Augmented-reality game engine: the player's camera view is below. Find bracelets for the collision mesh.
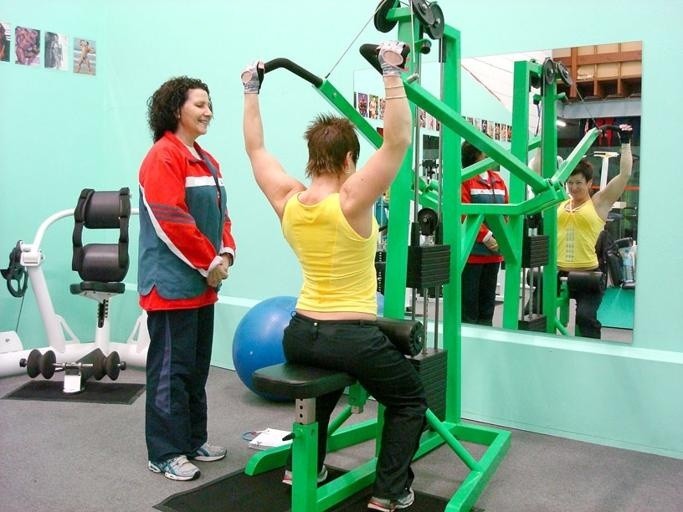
[384,95,407,100]
[385,84,402,89]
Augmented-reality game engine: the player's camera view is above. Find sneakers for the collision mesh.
[281,464,329,486]
[147,452,201,482]
[192,442,227,462]
[367,487,415,512]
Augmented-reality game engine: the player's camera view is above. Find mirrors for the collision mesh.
[353,40,642,347]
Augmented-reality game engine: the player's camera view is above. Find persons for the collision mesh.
[75,39,95,74]
[524,123,632,341]
[138,77,237,482]
[0,23,7,61]
[49,33,64,69]
[239,39,425,511]
[14,28,39,64]
[453,140,508,327]
